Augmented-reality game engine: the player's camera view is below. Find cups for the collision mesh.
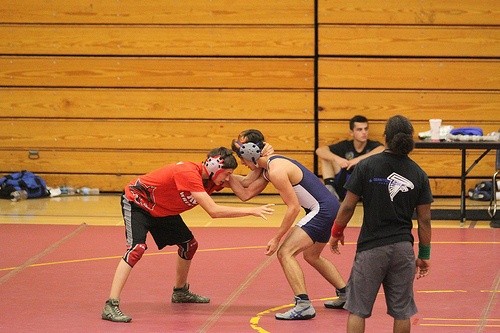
[429,119,442,141]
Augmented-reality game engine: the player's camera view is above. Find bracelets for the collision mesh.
[331,221,347,237]
[418,244,430,260]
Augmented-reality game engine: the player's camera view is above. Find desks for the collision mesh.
[415,140,500,223]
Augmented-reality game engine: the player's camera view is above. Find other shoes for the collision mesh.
[474,180,492,191]
[102,298,132,323]
[171,282,211,303]
[274,295,316,320]
[468,188,490,201]
[324,289,347,308]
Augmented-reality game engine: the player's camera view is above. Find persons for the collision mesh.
[101,142,275,323]
[314,114,386,202]
[333,115,433,333]
[227,129,348,319]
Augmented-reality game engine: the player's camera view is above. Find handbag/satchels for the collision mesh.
[0,169,51,199]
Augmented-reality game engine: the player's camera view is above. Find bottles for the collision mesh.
[56,187,75,195]
[76,187,99,195]
[8,189,28,201]
